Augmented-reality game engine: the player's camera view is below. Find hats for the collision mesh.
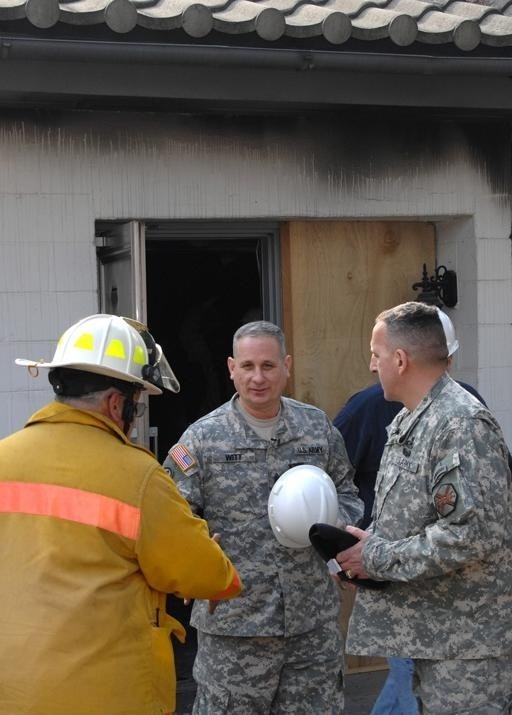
[309,524,392,590]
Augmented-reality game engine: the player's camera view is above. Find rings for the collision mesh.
[347,570,352,579]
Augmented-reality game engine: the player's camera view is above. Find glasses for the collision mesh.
[121,393,146,417]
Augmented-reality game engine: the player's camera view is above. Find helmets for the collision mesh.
[15,314,163,395]
[267,465,338,549]
[433,307,459,358]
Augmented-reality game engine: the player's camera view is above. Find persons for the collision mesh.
[337,301,512,714]
[333,305,490,715]
[160,322,365,715]
[0,314,244,715]
